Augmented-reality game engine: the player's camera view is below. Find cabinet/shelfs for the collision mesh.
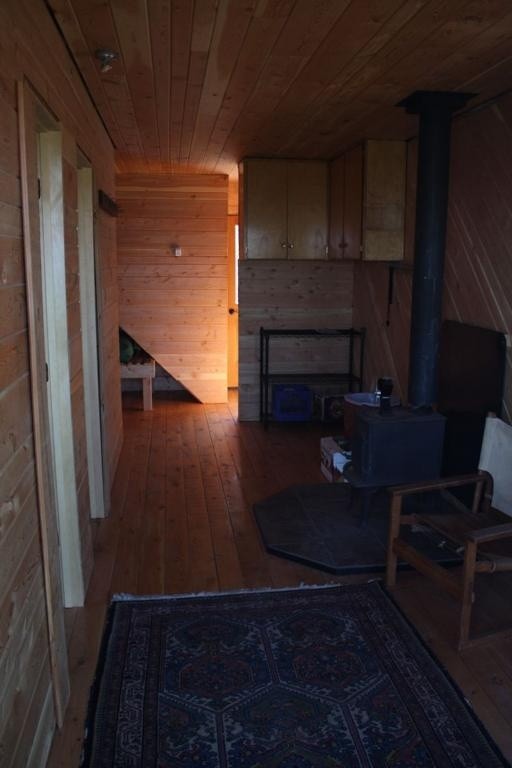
[257,325,366,431]
[330,137,408,262]
[238,156,330,259]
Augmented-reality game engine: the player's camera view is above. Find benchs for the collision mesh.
[121,356,156,411]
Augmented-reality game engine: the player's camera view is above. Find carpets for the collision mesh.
[78,576,509,767]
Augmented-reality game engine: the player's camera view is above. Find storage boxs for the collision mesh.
[319,436,352,482]
[313,389,345,424]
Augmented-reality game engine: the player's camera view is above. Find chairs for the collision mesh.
[383,411,512,651]
